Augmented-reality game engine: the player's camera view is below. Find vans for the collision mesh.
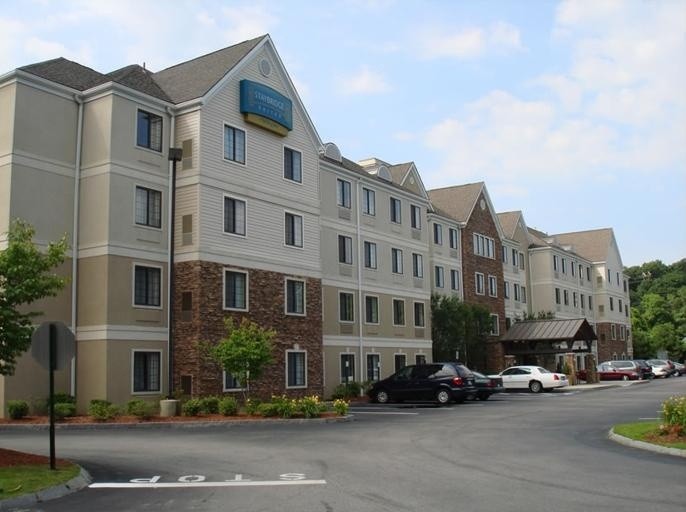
[366,361,478,407]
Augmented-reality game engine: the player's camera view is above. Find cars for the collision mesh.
[486,365,570,393]
[472,371,504,400]
[576,359,686,382]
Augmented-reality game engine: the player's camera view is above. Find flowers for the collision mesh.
[271,392,351,419]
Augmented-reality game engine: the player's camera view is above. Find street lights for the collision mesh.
[166,147,182,400]
